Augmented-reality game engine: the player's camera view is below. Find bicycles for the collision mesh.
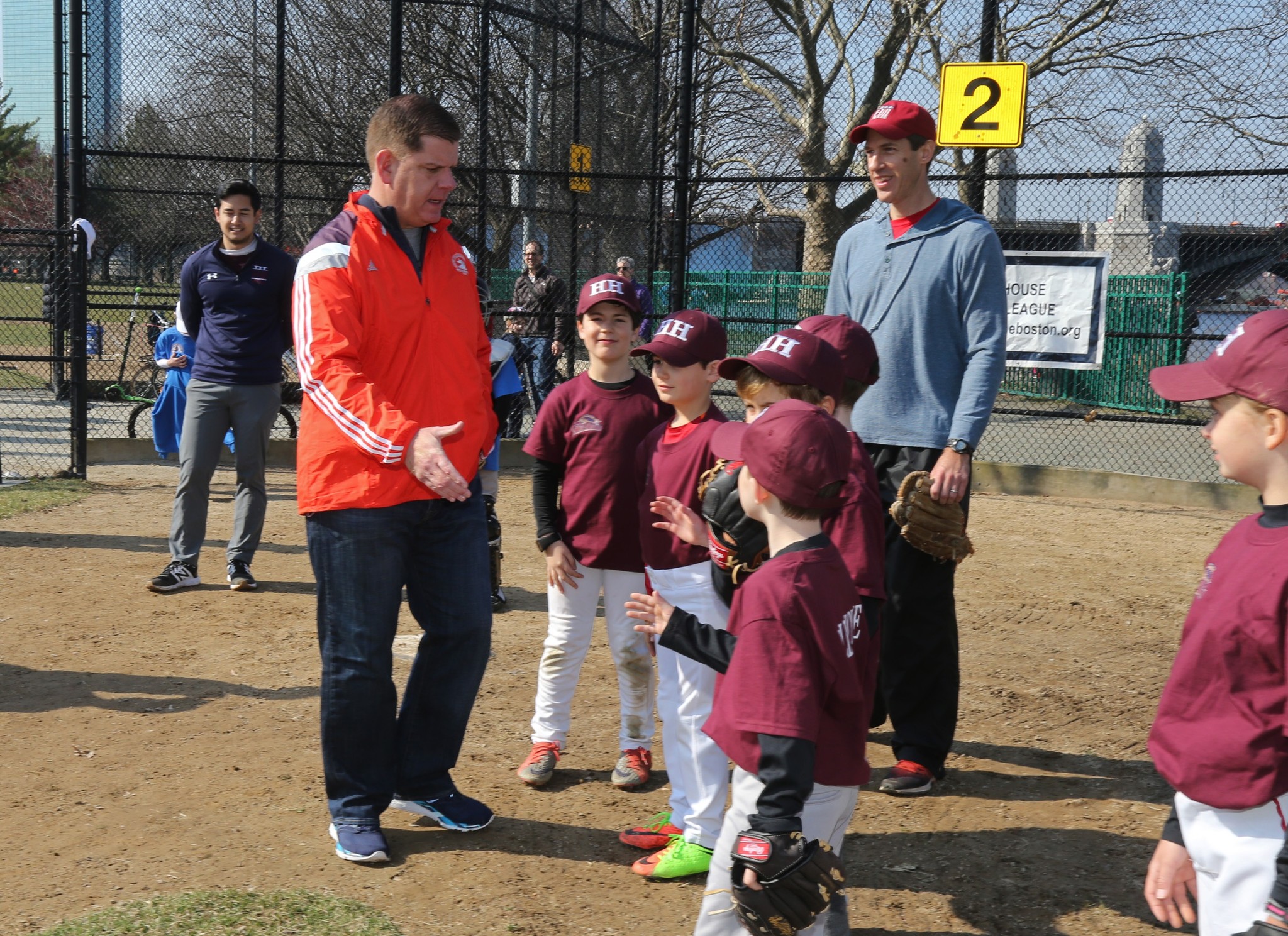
[127,302,297,438]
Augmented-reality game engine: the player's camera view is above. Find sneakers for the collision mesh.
[225,559,258,591]
[329,818,393,863]
[631,833,715,878]
[619,812,684,851]
[610,747,653,788]
[880,759,946,794]
[145,561,200,591]
[389,782,496,833]
[516,741,559,787]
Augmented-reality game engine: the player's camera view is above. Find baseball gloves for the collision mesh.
[730,829,844,936]
[891,469,975,566]
[702,459,769,607]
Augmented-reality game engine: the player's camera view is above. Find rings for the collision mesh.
[950,489,959,493]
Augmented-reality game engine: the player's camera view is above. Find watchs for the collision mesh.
[944,438,974,456]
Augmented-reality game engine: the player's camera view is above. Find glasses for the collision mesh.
[615,266,631,271]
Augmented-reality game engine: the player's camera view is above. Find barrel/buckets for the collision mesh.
[86,326,103,354]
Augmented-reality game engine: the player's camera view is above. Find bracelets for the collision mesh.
[537,532,561,553]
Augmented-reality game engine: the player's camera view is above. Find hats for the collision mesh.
[713,329,845,399]
[175,302,189,334]
[712,399,851,511]
[788,314,881,385]
[1147,308,1287,413]
[629,309,729,369]
[575,273,643,318]
[848,99,938,142]
[72,220,95,261]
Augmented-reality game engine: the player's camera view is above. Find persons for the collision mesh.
[1171,291,1200,365]
[506,241,571,438]
[822,99,1008,796]
[144,178,299,593]
[1143,804,1197,928]
[624,400,874,936]
[1147,308,1288,936]
[520,273,674,789]
[154,300,237,457]
[616,257,654,380]
[618,308,880,881]
[292,92,502,866]
